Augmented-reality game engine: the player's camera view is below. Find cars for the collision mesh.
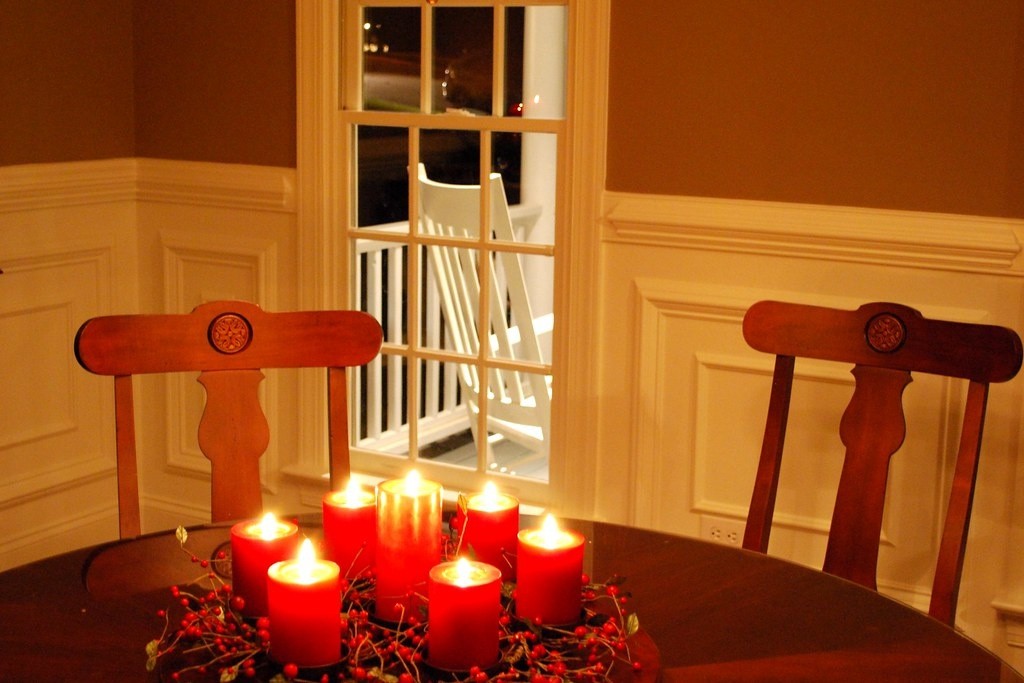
[441,46,524,117]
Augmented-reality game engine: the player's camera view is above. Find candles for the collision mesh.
[230,512,301,611]
[322,472,379,580]
[455,481,519,580]
[515,513,587,627]
[371,469,444,623]
[427,556,502,670]
[266,537,343,667]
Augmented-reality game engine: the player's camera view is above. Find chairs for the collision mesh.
[73,299,384,537]
[407,162,554,463]
[742,300,1024,625]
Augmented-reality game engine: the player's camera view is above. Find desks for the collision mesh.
[0,511,1024,683]
